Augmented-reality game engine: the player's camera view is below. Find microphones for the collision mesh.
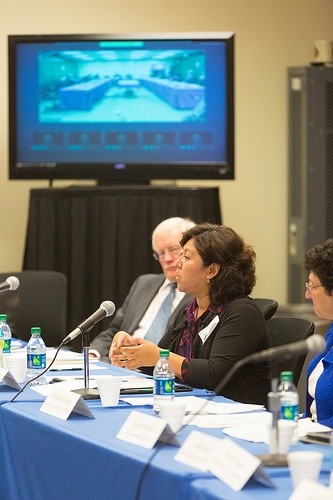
[62,301,116,344]
[240,334,326,369]
[0,276,20,292]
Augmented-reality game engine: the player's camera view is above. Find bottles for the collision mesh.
[0,314,12,369]
[153,349,175,415]
[0,327,5,369]
[275,372,299,445]
[27,327,46,386]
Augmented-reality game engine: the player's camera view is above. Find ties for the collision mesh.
[144,283,177,345]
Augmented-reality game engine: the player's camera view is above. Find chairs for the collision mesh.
[251,297,315,386]
[1,271,68,347]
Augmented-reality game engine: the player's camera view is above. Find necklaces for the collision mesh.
[196,307,199,319]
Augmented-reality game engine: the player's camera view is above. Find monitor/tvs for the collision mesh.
[8,34,235,187]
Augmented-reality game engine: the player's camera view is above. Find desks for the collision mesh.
[0,337,333,500]
[24,188,221,342]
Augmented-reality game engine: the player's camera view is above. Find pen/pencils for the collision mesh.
[49,368,82,371]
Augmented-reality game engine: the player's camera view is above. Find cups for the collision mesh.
[287,451,323,487]
[165,402,186,432]
[95,377,122,406]
[311,38,333,63]
[6,357,27,383]
[268,420,296,454]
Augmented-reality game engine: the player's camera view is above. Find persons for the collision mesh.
[107,222,276,412]
[84,217,202,381]
[302,235,333,430]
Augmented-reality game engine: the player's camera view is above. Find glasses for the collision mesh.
[153,246,181,260]
[306,283,329,294]
